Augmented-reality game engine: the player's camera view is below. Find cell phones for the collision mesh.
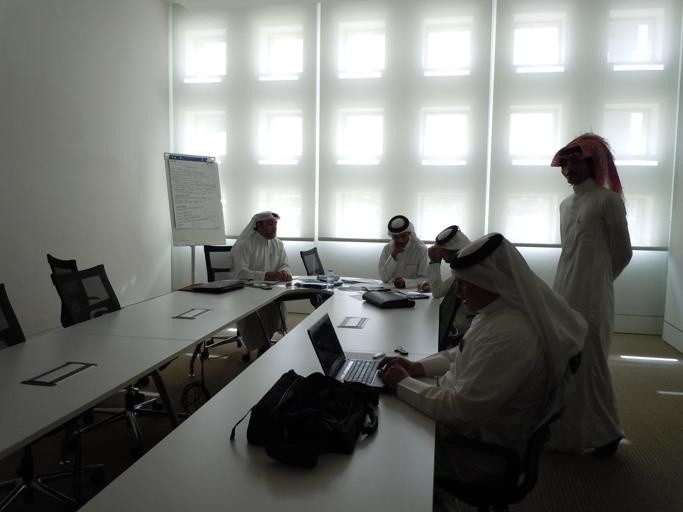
[408,294,429,299]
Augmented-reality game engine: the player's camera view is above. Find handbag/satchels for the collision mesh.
[246,369,381,454]
[362,290,414,308]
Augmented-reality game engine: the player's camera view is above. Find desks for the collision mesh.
[0,275,442,512]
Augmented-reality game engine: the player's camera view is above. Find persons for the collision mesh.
[549,133,632,456]
[230,212,292,357]
[430,226,473,299]
[378,215,429,289]
[376,233,587,511]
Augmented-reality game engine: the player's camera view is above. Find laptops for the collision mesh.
[307,313,388,391]
[192,280,244,293]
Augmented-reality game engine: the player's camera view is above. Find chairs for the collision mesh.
[189,243,250,374]
[45,251,190,440]
[298,247,331,306]
[0,279,83,511]
[435,345,583,510]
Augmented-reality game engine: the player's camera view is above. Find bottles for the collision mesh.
[326,269,334,291]
